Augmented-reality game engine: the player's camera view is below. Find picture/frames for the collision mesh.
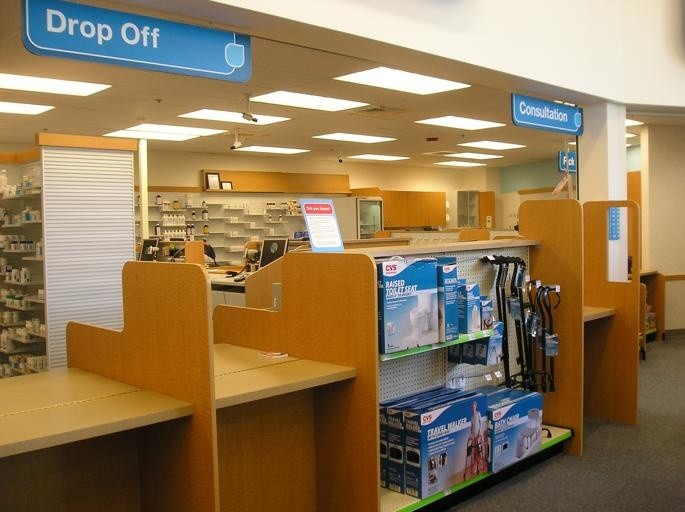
[221,181,233,189]
[206,172,221,190]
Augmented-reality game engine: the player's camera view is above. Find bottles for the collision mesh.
[0,169,47,377]
[137,194,302,241]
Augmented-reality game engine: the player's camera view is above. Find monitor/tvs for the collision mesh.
[139,238,158,261]
[259,238,289,270]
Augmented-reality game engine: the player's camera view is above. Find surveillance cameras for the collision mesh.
[242,113,258,122]
[336,153,343,164]
[230,141,242,149]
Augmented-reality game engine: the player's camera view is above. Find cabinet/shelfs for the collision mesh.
[0,132,138,378]
[0,191,640,511]
[628,270,666,358]
